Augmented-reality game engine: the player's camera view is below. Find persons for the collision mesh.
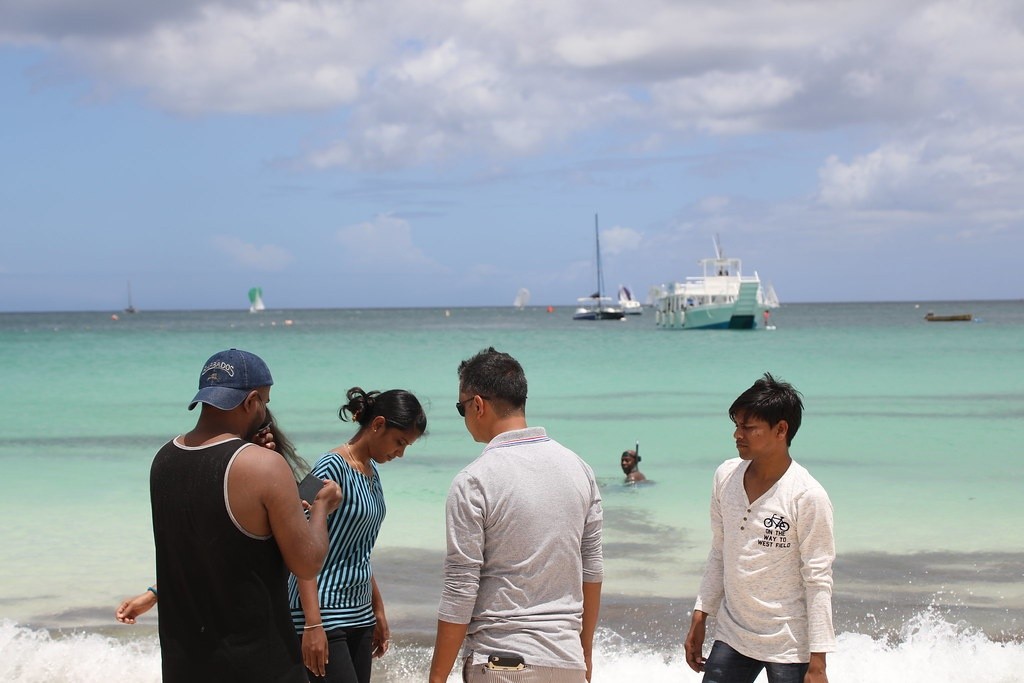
[684,372,837,683]
[720,265,728,276]
[763,310,769,326]
[429,347,603,683]
[116,347,344,683]
[286,387,427,683]
[620,450,646,483]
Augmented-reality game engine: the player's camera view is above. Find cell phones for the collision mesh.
[487,651,524,671]
[298,473,325,506]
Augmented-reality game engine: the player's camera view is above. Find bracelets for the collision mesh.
[304,622,324,629]
[148,586,157,595]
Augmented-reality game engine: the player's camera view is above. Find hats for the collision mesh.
[188,348,274,410]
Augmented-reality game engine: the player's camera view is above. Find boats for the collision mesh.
[925,312,974,322]
[654,235,780,330]
[616,285,644,316]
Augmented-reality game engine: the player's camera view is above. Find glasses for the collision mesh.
[456,396,491,417]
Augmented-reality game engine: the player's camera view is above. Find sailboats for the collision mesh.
[570,214,627,323]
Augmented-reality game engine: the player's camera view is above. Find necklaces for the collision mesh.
[345,442,373,476]
